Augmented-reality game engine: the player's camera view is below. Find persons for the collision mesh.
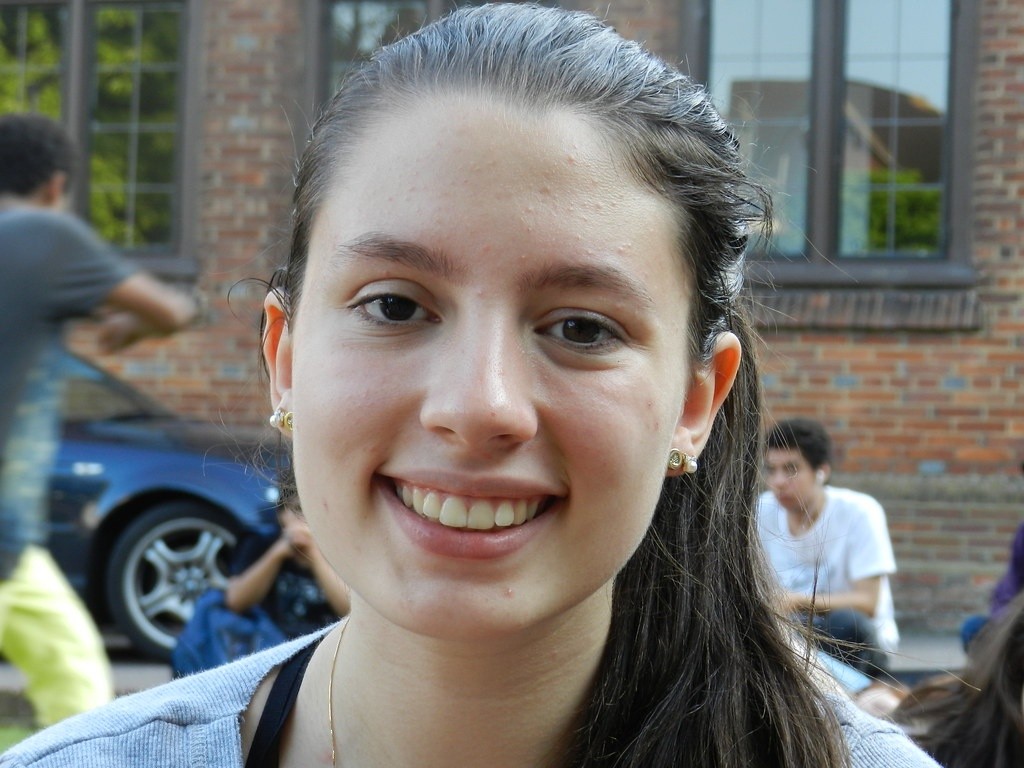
[1,2,944,768]
[746,420,900,676]
[0,107,196,736]
[895,453,1024,768]
[169,478,342,678]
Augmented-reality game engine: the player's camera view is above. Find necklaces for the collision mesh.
[327,618,349,766]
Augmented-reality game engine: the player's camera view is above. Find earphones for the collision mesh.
[815,471,826,491]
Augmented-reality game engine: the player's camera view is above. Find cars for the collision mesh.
[39,339,294,666]
